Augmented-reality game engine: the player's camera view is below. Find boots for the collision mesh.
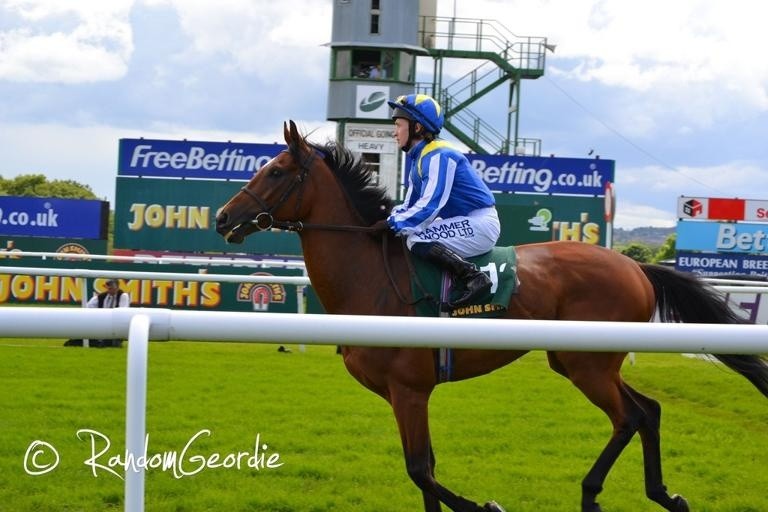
[422,242,493,307]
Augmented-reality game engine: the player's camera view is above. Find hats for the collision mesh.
[387,93,445,133]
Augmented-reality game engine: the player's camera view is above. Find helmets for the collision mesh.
[391,106,418,122]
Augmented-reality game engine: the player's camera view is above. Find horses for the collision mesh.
[214,120,767,512]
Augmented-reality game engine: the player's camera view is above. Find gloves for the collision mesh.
[368,219,392,236]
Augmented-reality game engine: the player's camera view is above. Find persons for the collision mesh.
[370,64,387,80]
[86,278,130,342]
[383,93,502,307]
